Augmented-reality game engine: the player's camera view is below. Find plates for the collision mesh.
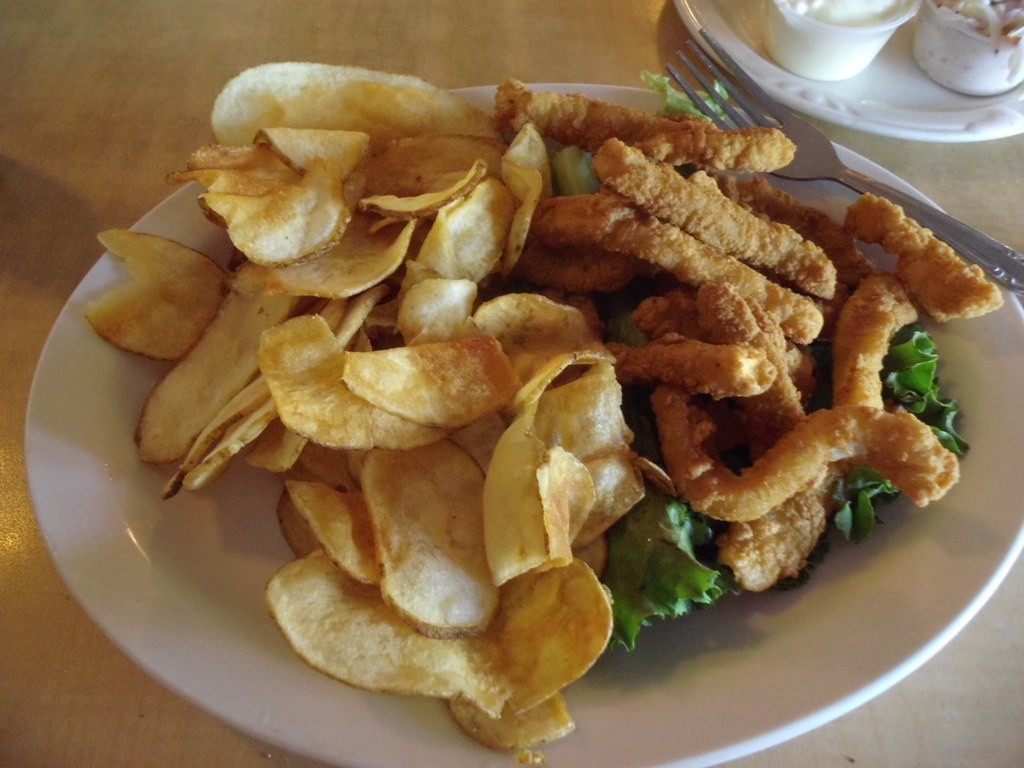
[675,0,1024,143]
[25,83,1024,768]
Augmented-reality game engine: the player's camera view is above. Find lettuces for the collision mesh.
[532,67,970,652]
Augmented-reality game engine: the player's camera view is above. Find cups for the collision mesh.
[763,0,923,82]
[912,0,1024,96]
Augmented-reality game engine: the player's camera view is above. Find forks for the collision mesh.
[664,27,1024,297]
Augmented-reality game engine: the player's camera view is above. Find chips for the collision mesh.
[85,60,647,757]
